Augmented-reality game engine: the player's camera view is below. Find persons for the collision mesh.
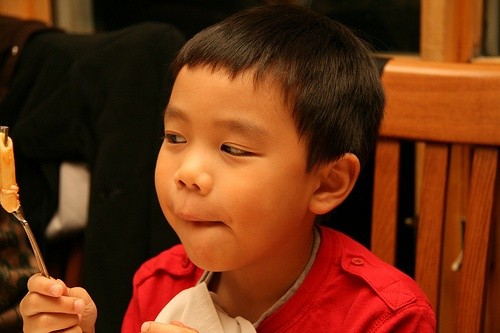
[19,2,437,332]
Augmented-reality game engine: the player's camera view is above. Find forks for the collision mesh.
[0,126,50,279]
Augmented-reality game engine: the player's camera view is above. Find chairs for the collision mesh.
[1,23,186,333]
[371,59,500,333]
